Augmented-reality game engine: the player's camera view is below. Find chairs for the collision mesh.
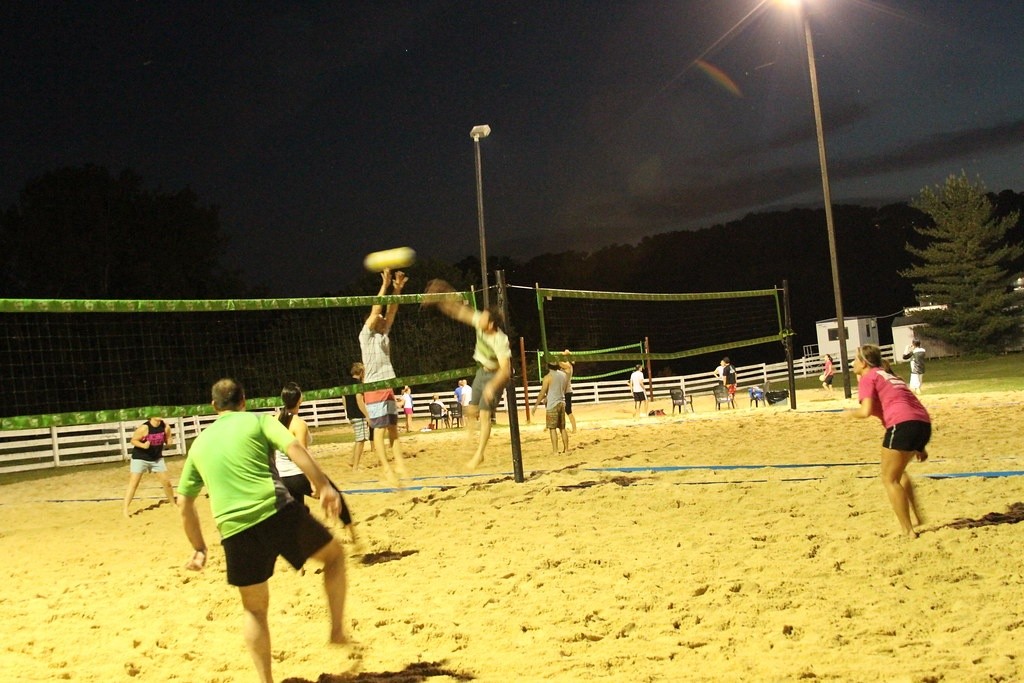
[429,403,450,430]
[712,385,734,412]
[449,401,464,428]
[750,381,771,407]
[670,388,695,415]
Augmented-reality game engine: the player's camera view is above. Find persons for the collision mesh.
[272,380,355,574]
[713,357,737,400]
[341,362,371,470]
[357,267,408,473]
[396,385,414,432]
[842,344,932,539]
[626,364,647,410]
[425,280,514,468]
[903,337,927,394]
[177,377,349,683]
[123,417,176,518]
[430,393,451,428]
[454,379,472,426]
[822,353,836,392]
[532,349,577,453]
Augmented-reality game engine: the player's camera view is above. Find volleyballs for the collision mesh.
[363,243,417,272]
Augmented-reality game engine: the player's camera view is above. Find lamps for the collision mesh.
[871,319,876,328]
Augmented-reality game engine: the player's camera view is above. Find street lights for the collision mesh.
[779,0,851,399]
[471,125,491,310]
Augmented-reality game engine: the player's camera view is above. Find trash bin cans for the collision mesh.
[766,389,789,406]
